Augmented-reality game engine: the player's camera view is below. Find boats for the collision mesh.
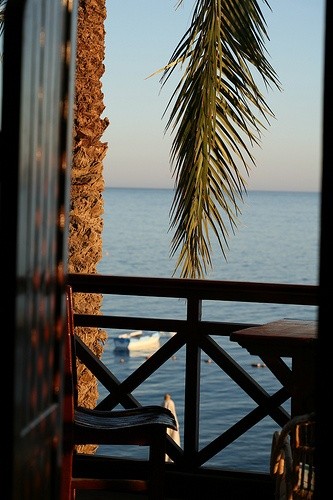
[113,330,160,349]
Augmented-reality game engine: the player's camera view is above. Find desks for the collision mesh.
[230,318,317,415]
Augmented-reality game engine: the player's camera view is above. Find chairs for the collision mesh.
[270,415,316,500]
[64,283,177,500]
